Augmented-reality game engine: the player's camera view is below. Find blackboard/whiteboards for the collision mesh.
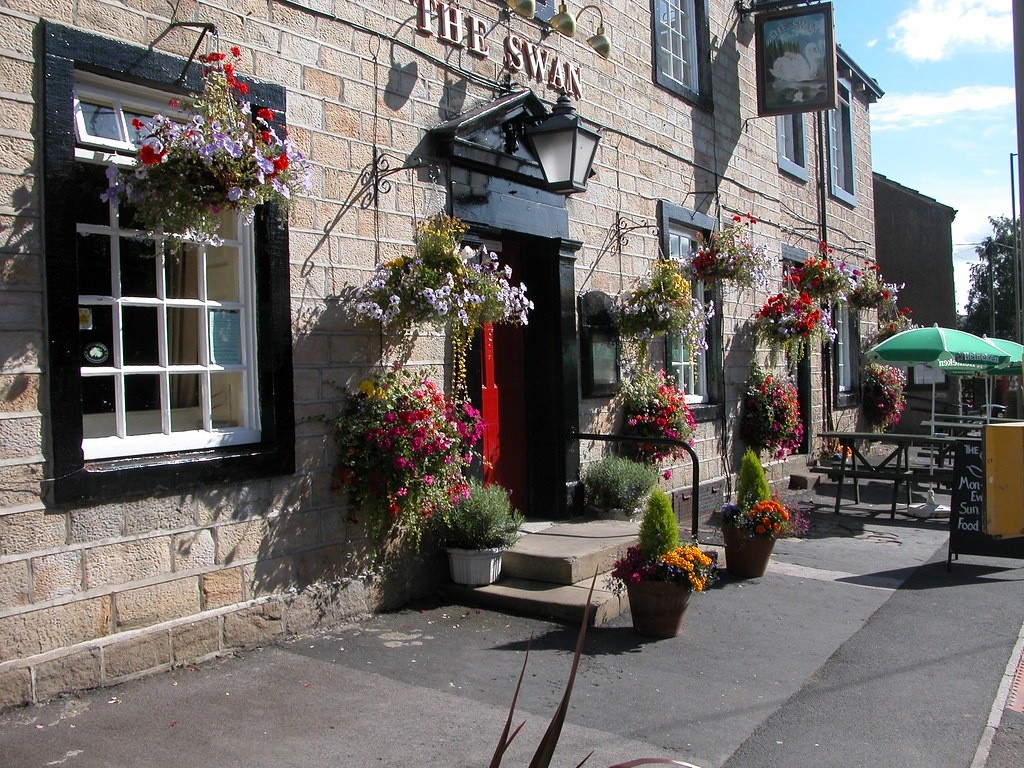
[950,440,1024,560]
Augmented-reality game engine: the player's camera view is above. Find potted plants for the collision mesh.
[423,474,527,585]
[577,454,662,520]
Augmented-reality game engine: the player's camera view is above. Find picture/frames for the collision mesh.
[755,1,838,118]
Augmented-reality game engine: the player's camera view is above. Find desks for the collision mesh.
[934,414,1023,437]
[920,418,984,467]
[818,430,982,518]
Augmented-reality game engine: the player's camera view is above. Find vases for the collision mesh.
[722,527,776,577]
[821,460,850,481]
[622,580,690,638]
[170,158,251,204]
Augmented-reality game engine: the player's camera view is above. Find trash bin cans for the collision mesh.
[980,404,1007,419]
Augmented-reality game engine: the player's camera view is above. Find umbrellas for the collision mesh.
[864,322,1024,518]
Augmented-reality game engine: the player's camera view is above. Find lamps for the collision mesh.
[517,86,602,194]
[503,0,611,59]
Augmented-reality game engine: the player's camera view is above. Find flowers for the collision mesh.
[607,213,924,479]
[601,488,720,596]
[713,446,814,547]
[99,47,314,263]
[295,213,516,591]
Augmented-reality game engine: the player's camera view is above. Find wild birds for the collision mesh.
[907,496,936,523]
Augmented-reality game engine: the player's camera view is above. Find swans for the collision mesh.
[768,42,819,83]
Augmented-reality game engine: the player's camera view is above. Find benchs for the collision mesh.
[809,447,958,482]
